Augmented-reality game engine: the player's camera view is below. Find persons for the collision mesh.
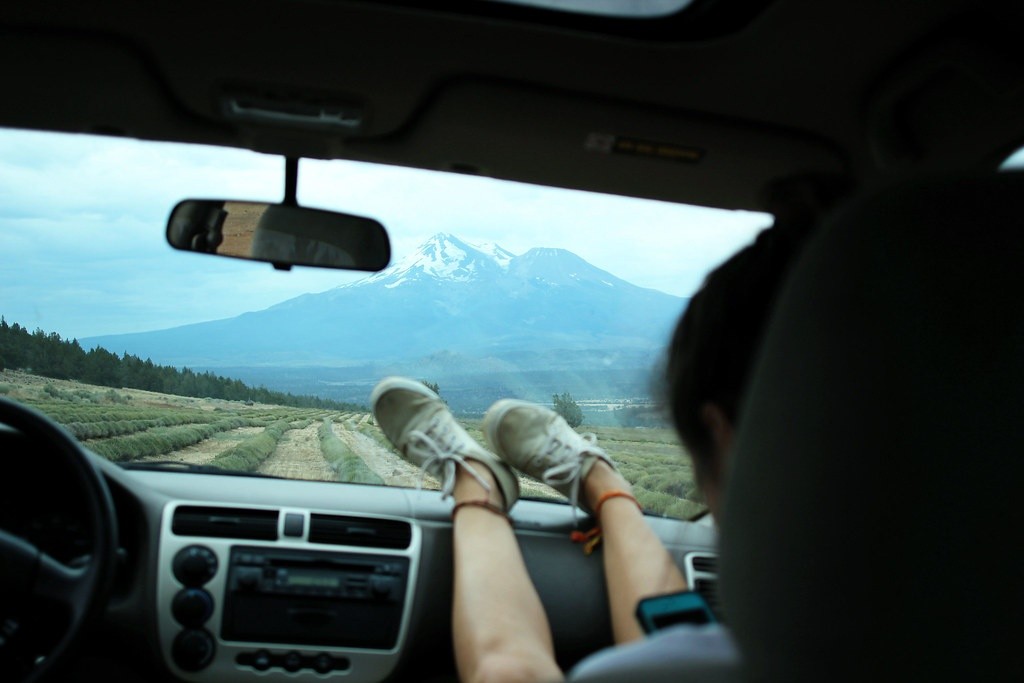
[371,218,822,683]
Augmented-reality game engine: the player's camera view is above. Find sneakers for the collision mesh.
[485,399,622,515]
[370,379,520,514]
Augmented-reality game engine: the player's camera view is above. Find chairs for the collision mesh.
[252,204,387,269]
[571,170,1024,683]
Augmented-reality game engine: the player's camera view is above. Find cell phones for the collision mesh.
[637,590,717,637]
